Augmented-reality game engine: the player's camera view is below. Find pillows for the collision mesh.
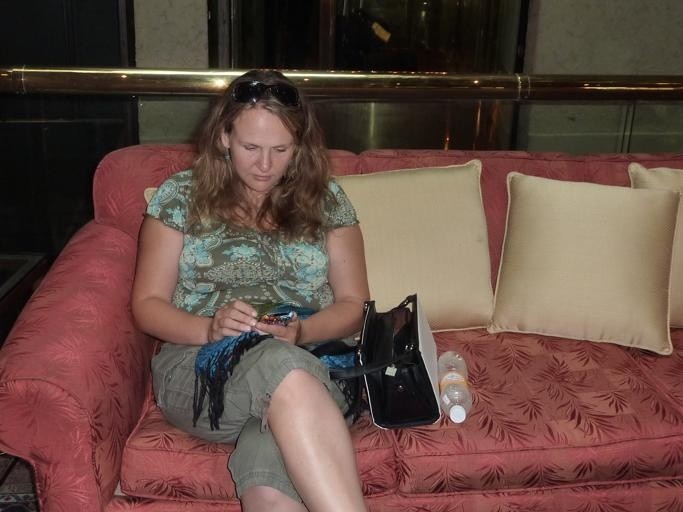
[488,171,680,355]
[329,160,494,333]
[627,162,681,328]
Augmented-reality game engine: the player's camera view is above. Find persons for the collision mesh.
[129,69,371,512]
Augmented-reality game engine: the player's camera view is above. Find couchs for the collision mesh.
[0,144,683,510]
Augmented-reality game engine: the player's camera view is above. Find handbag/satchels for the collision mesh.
[357,293,443,430]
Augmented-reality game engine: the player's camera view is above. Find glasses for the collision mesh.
[231,81,299,107]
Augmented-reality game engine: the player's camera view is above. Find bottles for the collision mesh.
[437,350,472,424]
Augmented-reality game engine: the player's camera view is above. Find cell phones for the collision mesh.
[259,312,294,328]
